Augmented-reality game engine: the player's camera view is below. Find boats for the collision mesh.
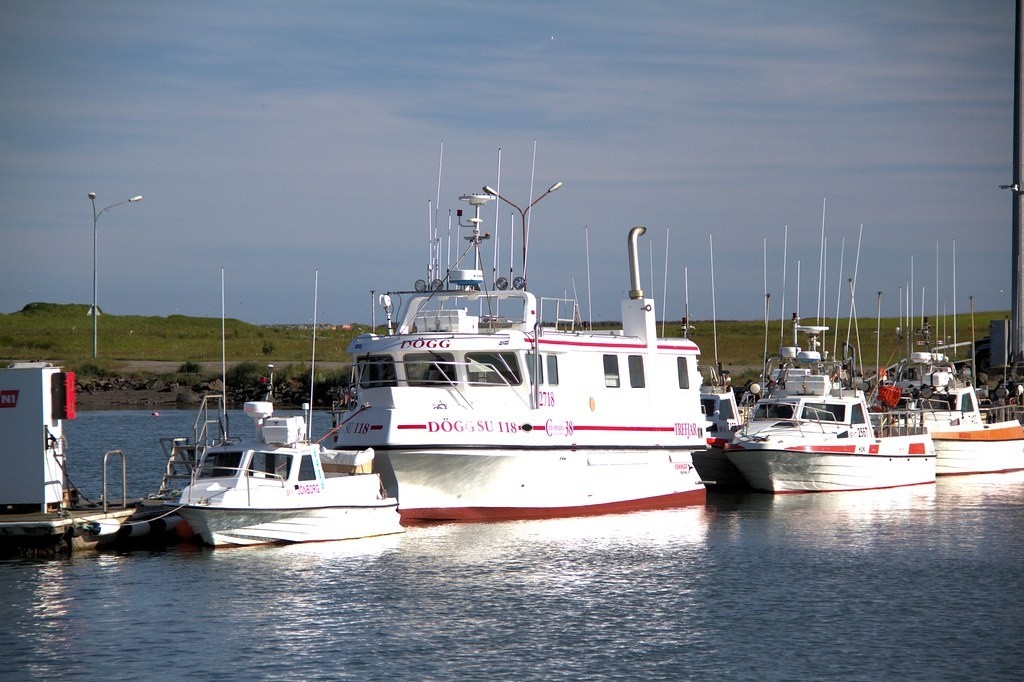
[336,195,716,521]
[892,386,1024,477]
[724,326,936,494]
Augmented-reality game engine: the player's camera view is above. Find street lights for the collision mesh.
[485,183,563,286]
[88,193,143,357]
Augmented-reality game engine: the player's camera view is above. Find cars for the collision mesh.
[177,418,406,551]
[969,336,1001,375]
